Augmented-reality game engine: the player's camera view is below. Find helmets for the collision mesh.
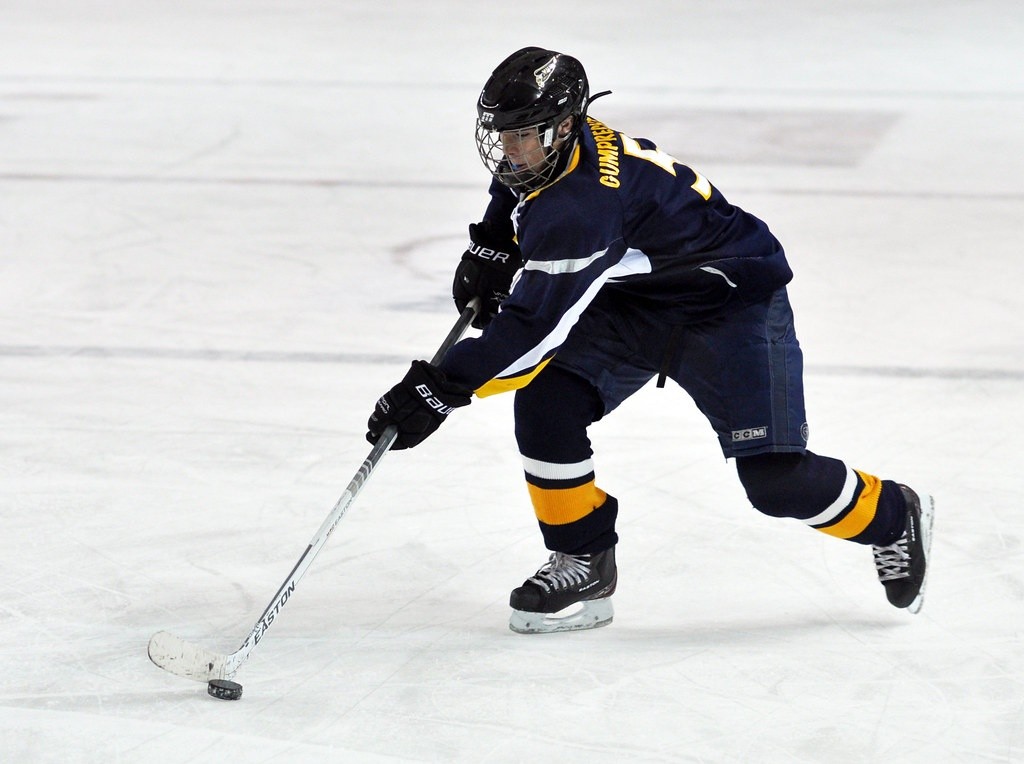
[475,46,589,191]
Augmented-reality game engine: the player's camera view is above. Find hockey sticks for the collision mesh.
[144,290,502,686]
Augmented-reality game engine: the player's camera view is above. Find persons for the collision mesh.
[366,47,934,634]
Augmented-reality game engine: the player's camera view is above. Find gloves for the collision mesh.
[453,224,523,329]
[366,360,473,450]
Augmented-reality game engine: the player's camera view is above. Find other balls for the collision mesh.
[207,679,243,701]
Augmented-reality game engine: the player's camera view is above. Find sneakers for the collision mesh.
[874,484,934,613]
[509,545,618,635]
